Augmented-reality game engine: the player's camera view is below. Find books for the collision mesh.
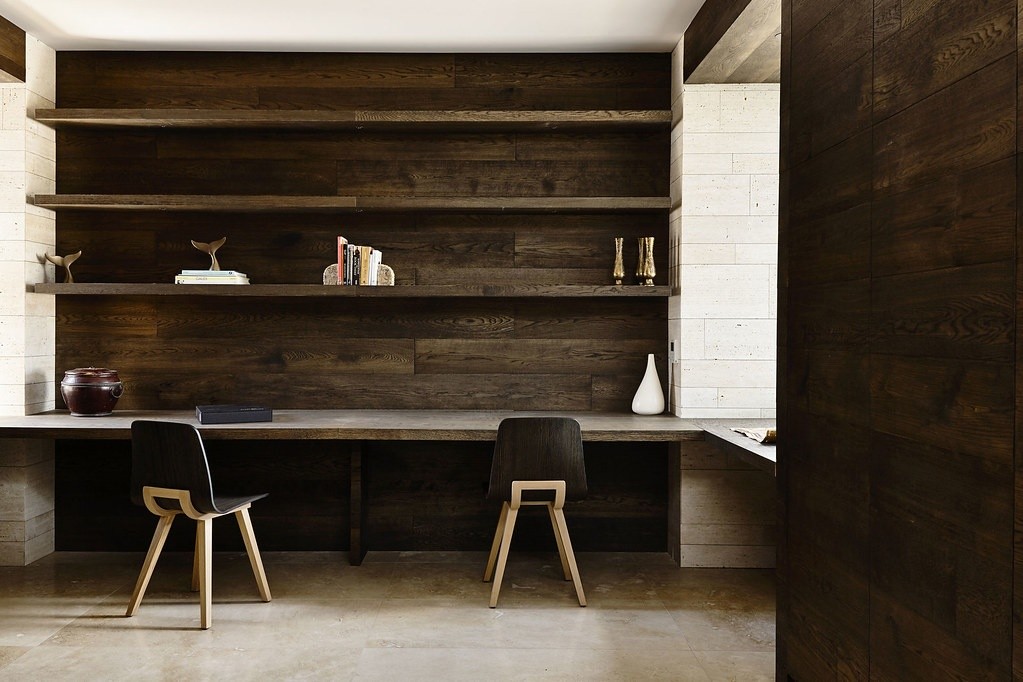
[174,270,250,284]
[195,406,272,425]
[338,237,382,285]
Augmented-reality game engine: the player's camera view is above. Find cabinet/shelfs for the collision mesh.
[33,107,675,298]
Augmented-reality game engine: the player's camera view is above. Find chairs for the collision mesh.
[482,416,588,607]
[125,421,272,630]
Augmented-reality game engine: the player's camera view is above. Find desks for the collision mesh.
[683,417,777,477]
[0,407,706,567]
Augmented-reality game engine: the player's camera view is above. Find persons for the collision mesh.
[355,247,360,255]
